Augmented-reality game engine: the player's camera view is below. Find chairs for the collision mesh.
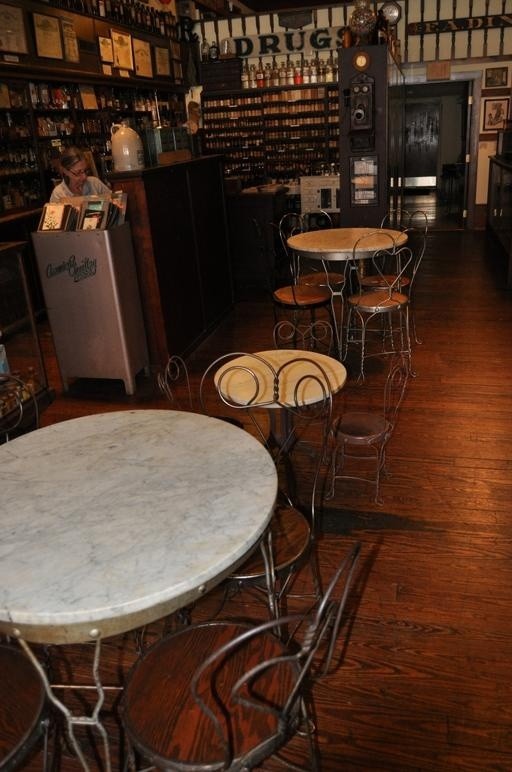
[2,349,362,771]
[156,321,411,519]
[274,210,428,384]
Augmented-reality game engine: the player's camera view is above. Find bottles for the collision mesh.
[0,117,118,213]
[46,0,179,40]
[209,41,219,61]
[240,57,339,89]
[201,40,210,63]
[220,39,232,59]
[211,147,337,182]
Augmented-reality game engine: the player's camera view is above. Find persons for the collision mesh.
[50,147,112,202]
[406,121,439,154]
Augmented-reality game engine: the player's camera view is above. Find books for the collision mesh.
[2,81,170,172]
[42,199,119,231]
[203,86,338,192]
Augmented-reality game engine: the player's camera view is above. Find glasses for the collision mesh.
[70,168,91,176]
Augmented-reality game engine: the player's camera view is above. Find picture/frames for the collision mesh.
[483,98,509,130]
[0,3,187,87]
[485,67,508,87]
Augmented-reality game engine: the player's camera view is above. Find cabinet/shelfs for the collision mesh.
[486,155,511,289]
[1,76,187,226]
[201,82,339,199]
[201,57,244,89]
[338,43,403,270]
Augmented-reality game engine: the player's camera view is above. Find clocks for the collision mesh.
[379,1,401,26]
